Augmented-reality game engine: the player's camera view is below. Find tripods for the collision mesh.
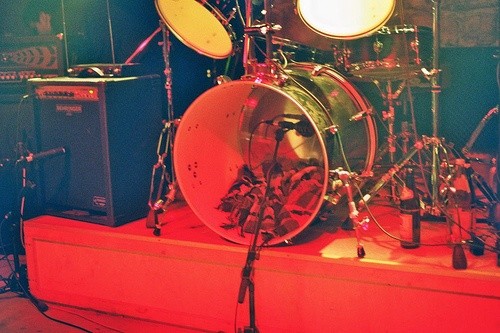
[342,1,499,231]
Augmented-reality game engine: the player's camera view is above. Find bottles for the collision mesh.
[399,165,421,248]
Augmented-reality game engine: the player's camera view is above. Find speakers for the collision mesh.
[27,71,164,228]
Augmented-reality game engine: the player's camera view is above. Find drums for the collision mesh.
[154,0,265,60]
[296,0,400,41]
[170,62,380,247]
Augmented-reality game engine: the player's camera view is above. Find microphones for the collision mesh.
[268,119,314,137]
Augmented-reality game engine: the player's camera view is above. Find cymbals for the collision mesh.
[353,67,420,82]
